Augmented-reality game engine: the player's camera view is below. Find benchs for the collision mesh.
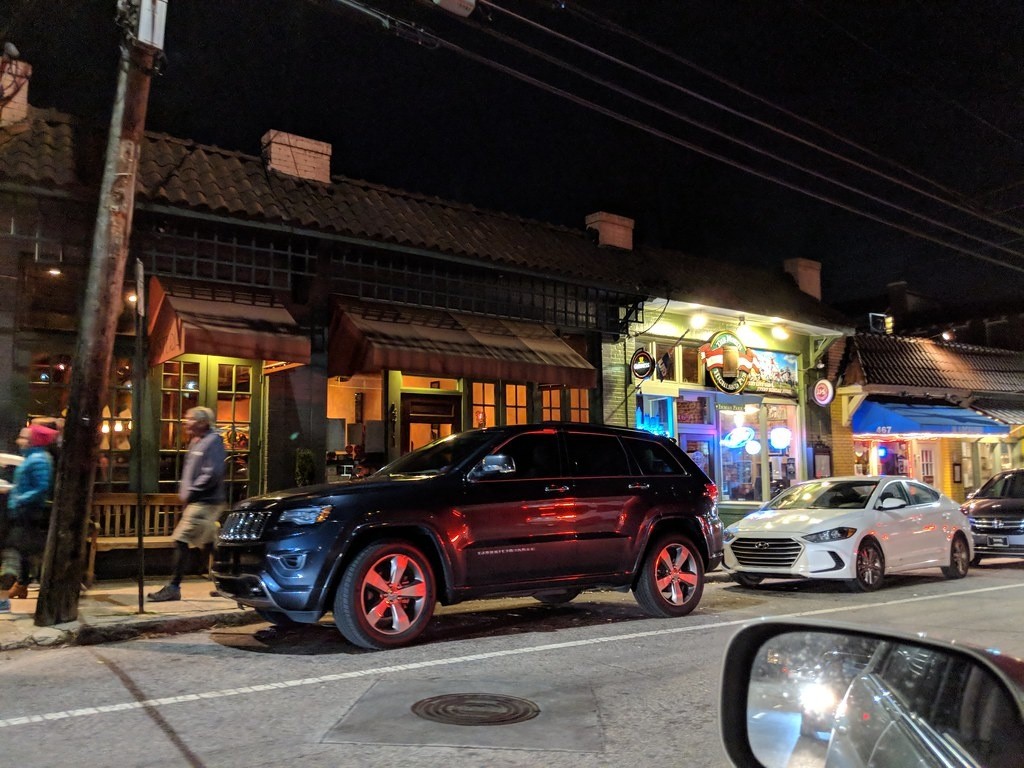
[82,492,227,584]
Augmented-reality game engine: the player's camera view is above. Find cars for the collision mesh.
[720,622,1024,768]
[723,474,975,593]
[795,652,873,737]
[959,467,1024,566]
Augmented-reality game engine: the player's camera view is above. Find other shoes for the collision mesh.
[8,582,28,599]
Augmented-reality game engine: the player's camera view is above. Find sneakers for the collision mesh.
[210,591,222,597]
[148,583,181,601]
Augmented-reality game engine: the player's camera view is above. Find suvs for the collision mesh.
[207,421,726,650]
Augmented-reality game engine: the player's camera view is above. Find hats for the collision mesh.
[28,424,59,446]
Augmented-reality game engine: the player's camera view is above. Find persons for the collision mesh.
[0,424,60,613]
[7,416,59,600]
[146,405,226,602]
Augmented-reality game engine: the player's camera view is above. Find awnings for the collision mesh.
[326,300,599,391]
[850,394,1011,439]
[968,400,1024,426]
[145,275,313,375]
